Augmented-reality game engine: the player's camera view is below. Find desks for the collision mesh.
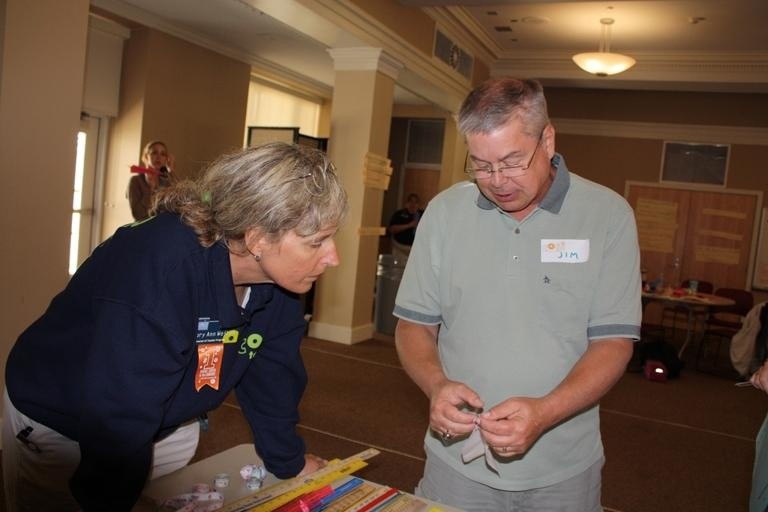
[642,282,736,362]
[141,442,466,512]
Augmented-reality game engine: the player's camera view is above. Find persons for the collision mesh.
[393,74,643,512]
[6,142,346,511]
[748,304,768,511]
[125,142,178,221]
[388,193,424,255]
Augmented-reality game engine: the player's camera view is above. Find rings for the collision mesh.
[503,447,507,453]
[443,431,451,441]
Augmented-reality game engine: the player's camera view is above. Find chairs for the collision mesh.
[659,278,754,371]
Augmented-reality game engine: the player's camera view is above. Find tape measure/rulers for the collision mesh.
[214,447,428,512]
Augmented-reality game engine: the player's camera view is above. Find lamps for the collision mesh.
[571,18,637,76]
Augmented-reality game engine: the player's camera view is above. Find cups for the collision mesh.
[689,280,698,293]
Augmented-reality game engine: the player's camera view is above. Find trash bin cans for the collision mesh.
[376,252,407,337]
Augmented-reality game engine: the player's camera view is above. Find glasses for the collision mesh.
[462,128,546,179]
[280,153,337,197]
